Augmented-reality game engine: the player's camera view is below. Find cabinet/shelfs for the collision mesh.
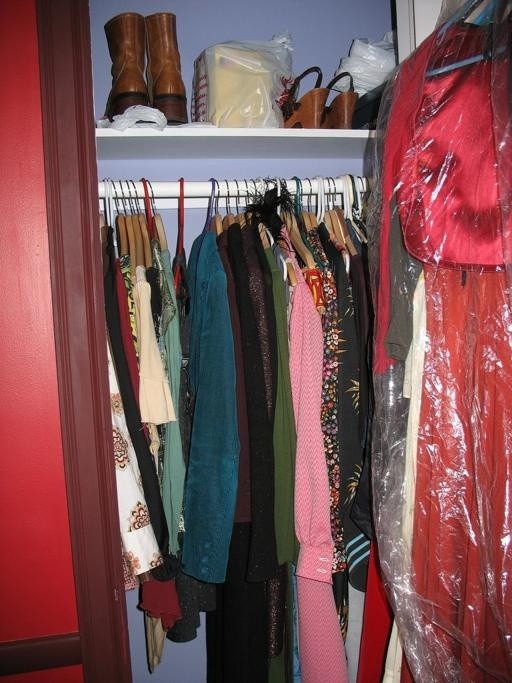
[0,2,448,682]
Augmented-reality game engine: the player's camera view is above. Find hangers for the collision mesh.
[96,174,370,289]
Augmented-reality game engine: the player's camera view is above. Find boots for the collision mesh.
[103,11,188,124]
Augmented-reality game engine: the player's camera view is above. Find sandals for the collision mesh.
[281,66,359,129]
